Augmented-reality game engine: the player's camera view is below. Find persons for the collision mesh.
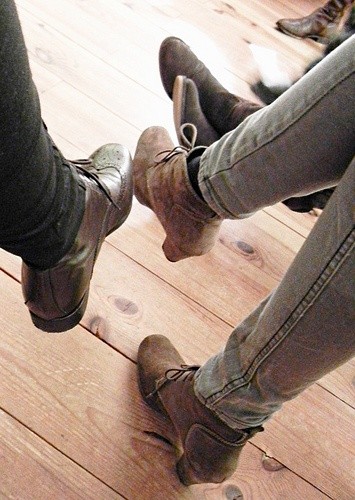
[133,31,355,486]
[160,0,355,215]
[0,0,133,334]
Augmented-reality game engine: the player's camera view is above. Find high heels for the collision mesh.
[131,126,223,262]
[136,334,264,487]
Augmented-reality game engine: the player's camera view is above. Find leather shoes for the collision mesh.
[159,37,236,150]
[21,143,132,332]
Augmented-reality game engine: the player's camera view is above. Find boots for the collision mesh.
[275,0,347,45]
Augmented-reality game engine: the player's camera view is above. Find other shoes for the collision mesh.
[250,80,290,106]
